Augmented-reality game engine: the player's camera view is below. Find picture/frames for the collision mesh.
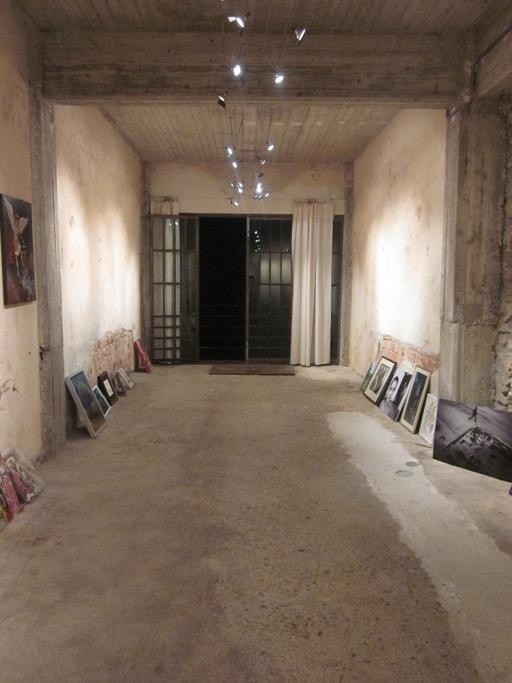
[0,192,38,308]
[360,353,439,445]
[63,362,137,438]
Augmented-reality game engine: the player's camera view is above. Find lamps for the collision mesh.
[213,0,311,206]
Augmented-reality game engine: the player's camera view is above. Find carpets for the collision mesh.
[209,362,296,375]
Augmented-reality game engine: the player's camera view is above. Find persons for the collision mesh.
[379,375,399,421]
